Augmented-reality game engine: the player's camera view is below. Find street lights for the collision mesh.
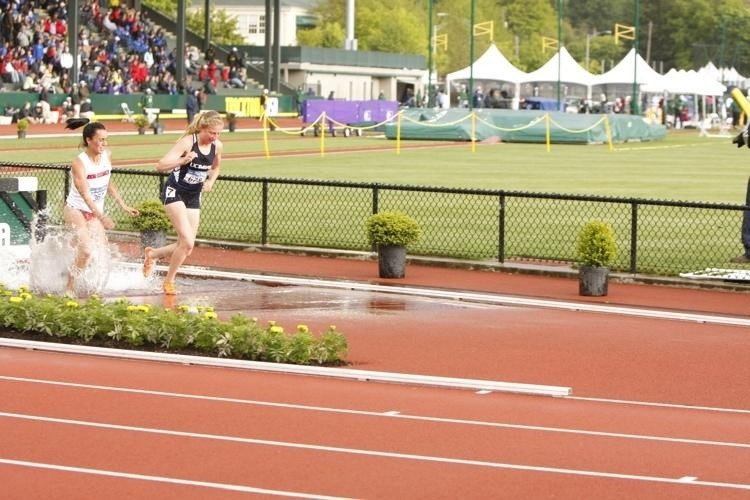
[432,11,446,76]
[583,28,614,72]
[503,21,520,69]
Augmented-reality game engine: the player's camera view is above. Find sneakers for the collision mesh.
[163,278,175,295]
[143,248,153,278]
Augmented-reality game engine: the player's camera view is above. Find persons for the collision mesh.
[258,89,270,122]
[0,0,251,126]
[730,120,750,263]
[295,84,304,114]
[62,116,141,300]
[143,109,225,295]
[728,99,743,126]
[402,83,721,131]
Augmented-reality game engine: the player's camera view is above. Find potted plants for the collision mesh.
[364,210,421,278]
[128,200,176,259]
[572,217,618,296]
